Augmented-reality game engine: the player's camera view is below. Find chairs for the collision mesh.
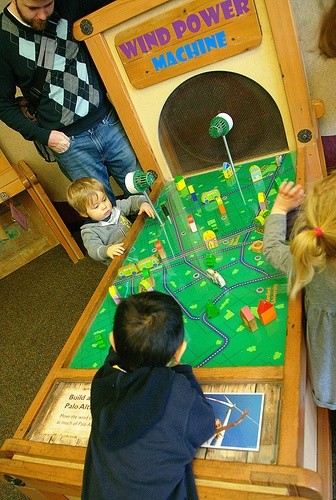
[0,149,85,279]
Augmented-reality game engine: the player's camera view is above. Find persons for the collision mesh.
[263,161,336,456]
[0,0,148,208]
[214,409,248,438]
[80,291,215,500]
[67,177,155,266]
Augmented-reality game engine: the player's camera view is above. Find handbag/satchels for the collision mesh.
[16,95,56,163]
